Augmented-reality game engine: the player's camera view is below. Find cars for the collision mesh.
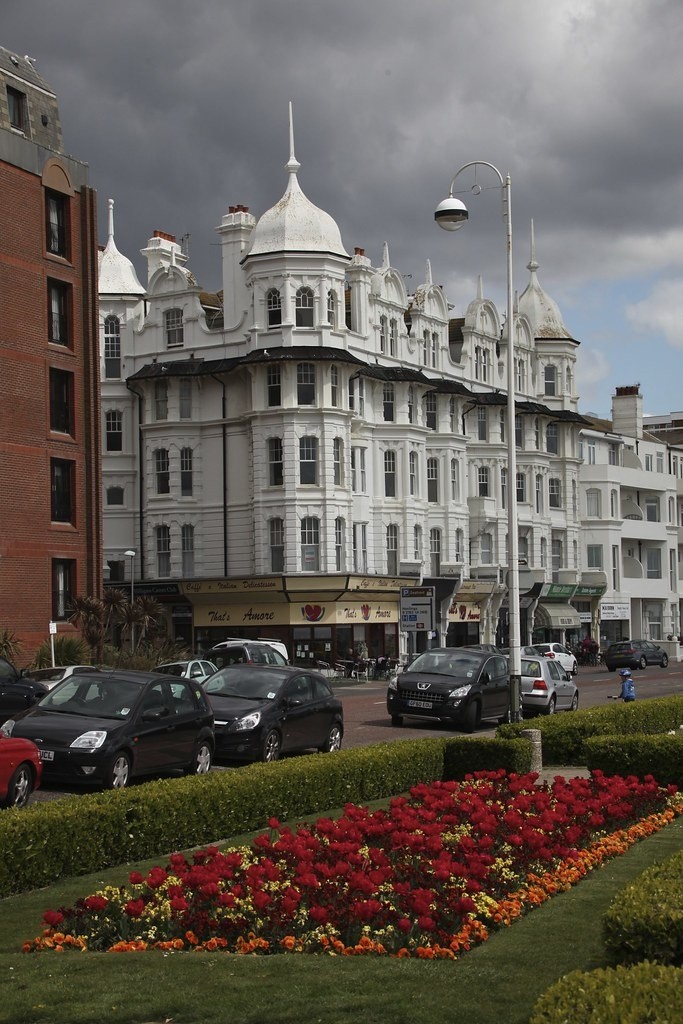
[0,654,50,724]
[520,656,579,715]
[199,664,344,764]
[529,643,579,676]
[498,646,541,658]
[1,669,216,793]
[28,664,107,706]
[461,644,502,654]
[0,736,43,810]
[148,660,225,699]
[386,647,510,733]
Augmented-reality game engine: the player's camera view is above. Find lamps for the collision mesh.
[263,350,270,358]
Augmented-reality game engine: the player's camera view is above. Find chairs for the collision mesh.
[316,659,407,683]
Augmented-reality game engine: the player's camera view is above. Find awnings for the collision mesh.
[534,603,581,630]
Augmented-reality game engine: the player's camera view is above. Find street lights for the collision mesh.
[432,159,522,723]
[123,550,136,656]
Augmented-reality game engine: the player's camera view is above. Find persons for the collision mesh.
[583,635,600,665]
[618,669,635,702]
[345,648,355,678]
[378,655,390,677]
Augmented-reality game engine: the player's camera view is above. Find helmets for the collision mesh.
[618,669,631,676]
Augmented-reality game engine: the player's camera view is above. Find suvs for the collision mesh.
[203,641,289,672]
[606,639,669,672]
